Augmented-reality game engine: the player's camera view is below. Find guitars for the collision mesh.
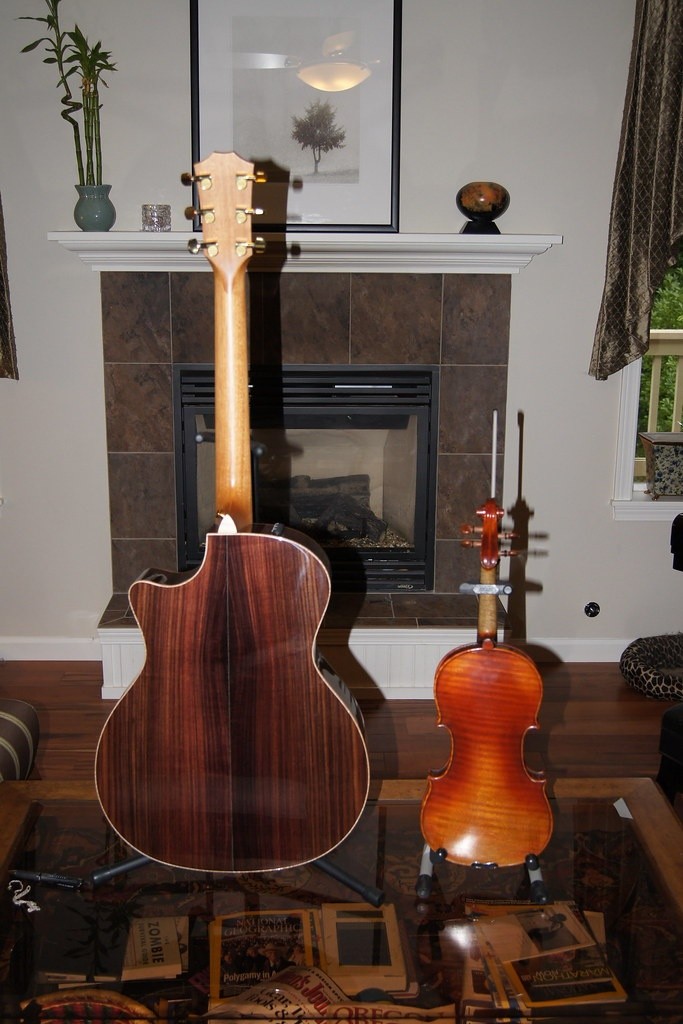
[76,152,384,906]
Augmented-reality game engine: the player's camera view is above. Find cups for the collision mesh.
[142,205,171,232]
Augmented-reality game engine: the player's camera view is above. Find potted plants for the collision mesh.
[15,0,117,230]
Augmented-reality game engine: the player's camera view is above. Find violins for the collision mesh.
[415,408,554,902]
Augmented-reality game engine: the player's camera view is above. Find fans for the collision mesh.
[207,28,399,79]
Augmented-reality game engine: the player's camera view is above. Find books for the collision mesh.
[36,902,628,1024]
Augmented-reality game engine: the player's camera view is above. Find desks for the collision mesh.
[0,776,683,1024]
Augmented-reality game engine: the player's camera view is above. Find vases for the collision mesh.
[639,432,683,500]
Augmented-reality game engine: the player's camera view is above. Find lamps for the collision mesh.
[297,60,372,93]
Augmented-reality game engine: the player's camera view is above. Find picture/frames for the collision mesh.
[190,0,404,234]
